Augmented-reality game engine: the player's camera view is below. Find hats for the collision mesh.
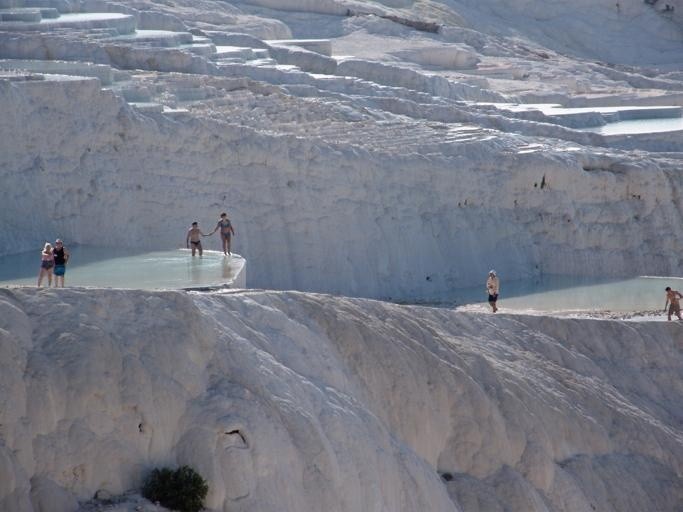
[488,269,497,277]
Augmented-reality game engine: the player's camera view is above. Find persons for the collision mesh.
[661,286,682,321]
[37,242,53,287]
[486,270,499,312]
[51,238,66,288]
[209,211,234,257]
[185,221,209,256]
